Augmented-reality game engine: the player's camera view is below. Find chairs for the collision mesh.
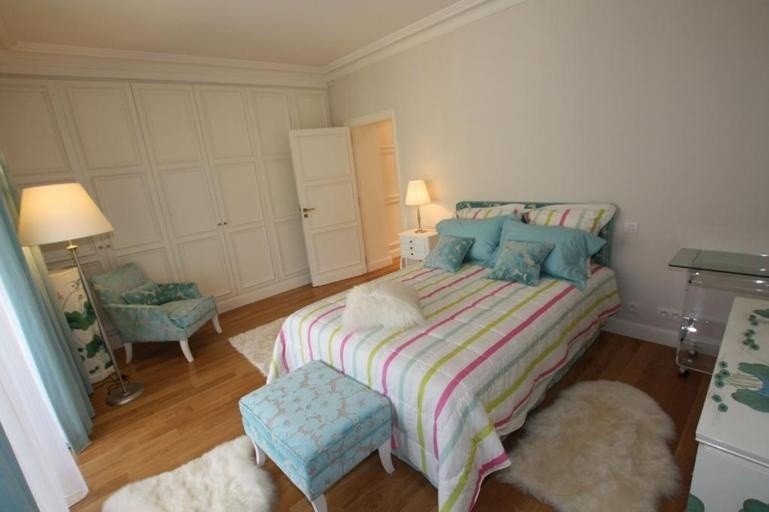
[89,263,223,363]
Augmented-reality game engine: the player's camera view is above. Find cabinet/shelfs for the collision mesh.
[1,76,183,353]
[247,78,339,292]
[685,293,769,512]
[130,78,286,318]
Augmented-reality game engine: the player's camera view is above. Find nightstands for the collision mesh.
[398,228,439,270]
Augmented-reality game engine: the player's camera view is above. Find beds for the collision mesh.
[265,200,622,512]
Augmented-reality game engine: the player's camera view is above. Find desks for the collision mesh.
[667,247,769,379]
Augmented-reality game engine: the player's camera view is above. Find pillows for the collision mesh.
[424,204,618,291]
[339,275,425,334]
[120,278,166,307]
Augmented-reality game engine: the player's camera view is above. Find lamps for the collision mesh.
[405,180,432,234]
[16,182,146,406]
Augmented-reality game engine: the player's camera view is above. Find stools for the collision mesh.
[238,361,396,511]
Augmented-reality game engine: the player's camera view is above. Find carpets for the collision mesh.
[228,316,288,377]
[494,380,685,511]
[100,434,279,511]
